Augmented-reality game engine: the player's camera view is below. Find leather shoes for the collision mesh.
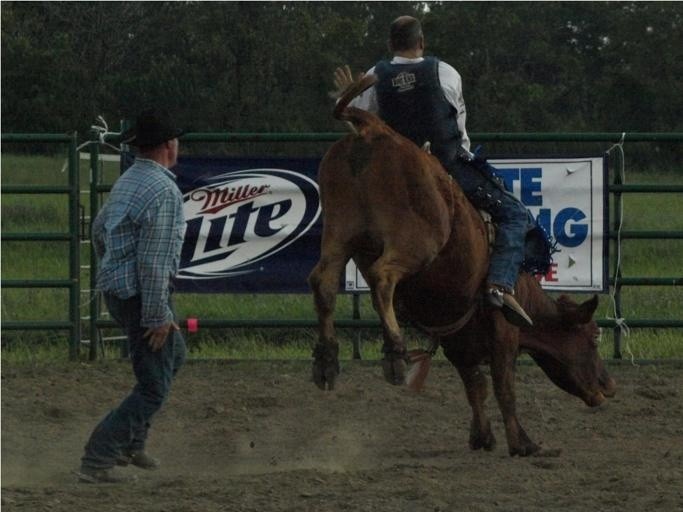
[123,449,161,471]
[484,283,532,329]
[79,470,138,485]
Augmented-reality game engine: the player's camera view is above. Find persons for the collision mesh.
[333,16,535,330]
[81,111,193,483]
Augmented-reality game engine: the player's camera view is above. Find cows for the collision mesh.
[307,64,617,457]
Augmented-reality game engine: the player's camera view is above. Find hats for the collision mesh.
[125,109,184,145]
